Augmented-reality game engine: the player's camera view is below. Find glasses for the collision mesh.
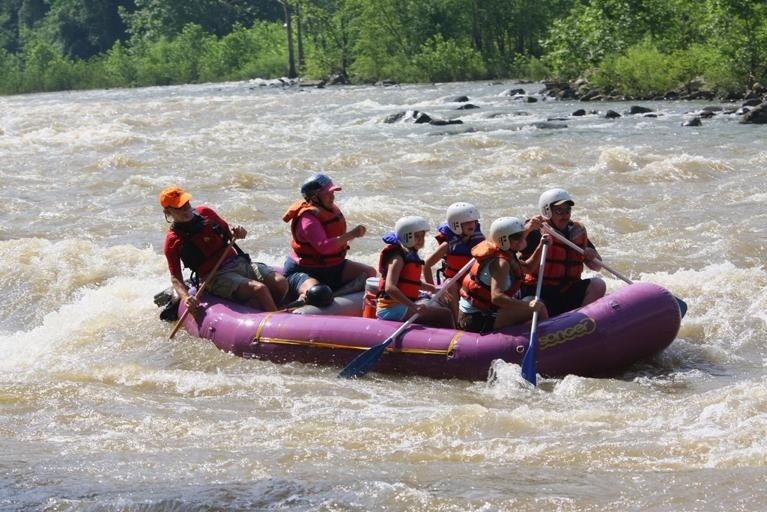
[553,208,570,215]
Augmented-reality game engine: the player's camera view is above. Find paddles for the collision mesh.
[536,222,687,317]
[338,259,477,378]
[522,238,548,384]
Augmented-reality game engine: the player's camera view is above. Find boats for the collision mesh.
[163,263,686,382]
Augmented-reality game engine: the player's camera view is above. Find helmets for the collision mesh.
[300,174,331,202]
[538,189,575,221]
[490,217,525,251]
[306,284,334,306]
[396,216,430,248]
[445,202,478,234]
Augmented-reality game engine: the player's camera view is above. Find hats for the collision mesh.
[159,188,191,208]
[319,180,341,193]
[553,199,574,206]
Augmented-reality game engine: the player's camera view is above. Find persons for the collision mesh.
[159,187,289,314]
[375,189,606,332]
[282,174,377,307]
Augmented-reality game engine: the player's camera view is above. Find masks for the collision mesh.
[168,209,195,223]
